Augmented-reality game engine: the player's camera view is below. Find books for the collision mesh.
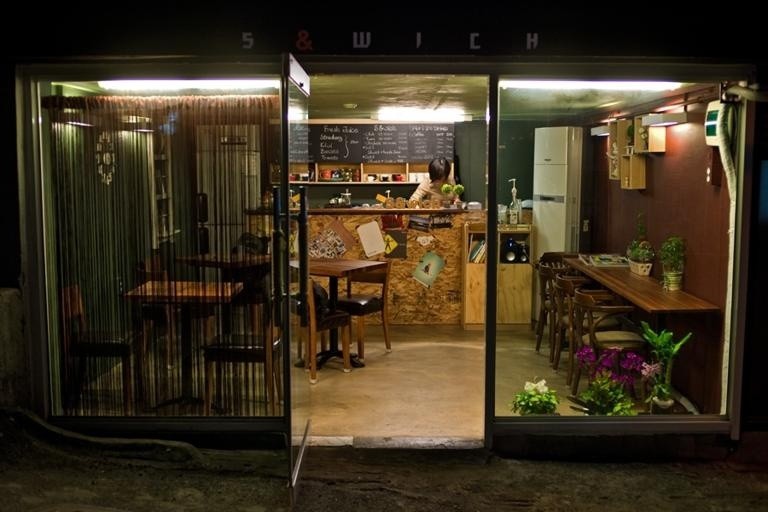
[590,253,630,268]
[468,234,487,263]
[577,252,597,267]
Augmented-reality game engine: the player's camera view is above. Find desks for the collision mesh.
[298,257,381,371]
[124,280,240,415]
[178,250,270,344]
[562,252,721,413]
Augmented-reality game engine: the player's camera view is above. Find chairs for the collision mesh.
[572,288,649,400]
[290,266,350,384]
[551,274,620,372]
[60,284,134,411]
[202,293,286,421]
[139,252,193,356]
[533,262,588,356]
[330,257,395,364]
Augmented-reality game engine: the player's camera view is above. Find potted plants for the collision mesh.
[441,183,453,208]
[658,237,686,293]
[627,235,653,277]
[452,184,466,208]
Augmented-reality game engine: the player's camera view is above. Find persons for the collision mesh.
[408,157,462,205]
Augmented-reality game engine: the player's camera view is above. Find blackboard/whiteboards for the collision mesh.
[269,124,455,163]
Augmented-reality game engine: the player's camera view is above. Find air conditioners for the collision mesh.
[531,127,580,326]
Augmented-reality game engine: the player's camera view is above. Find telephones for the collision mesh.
[197,193,208,223]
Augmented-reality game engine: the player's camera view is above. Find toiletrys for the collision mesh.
[508,178,522,224]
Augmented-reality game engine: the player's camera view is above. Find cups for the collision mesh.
[292,173,300,181]
[343,193,352,205]
[320,169,331,179]
[353,169,360,182]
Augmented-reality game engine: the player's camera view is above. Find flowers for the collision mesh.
[510,375,560,415]
[637,360,665,386]
[574,342,643,387]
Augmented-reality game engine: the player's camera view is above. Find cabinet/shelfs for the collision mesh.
[145,120,177,246]
[460,220,532,329]
[606,119,666,192]
